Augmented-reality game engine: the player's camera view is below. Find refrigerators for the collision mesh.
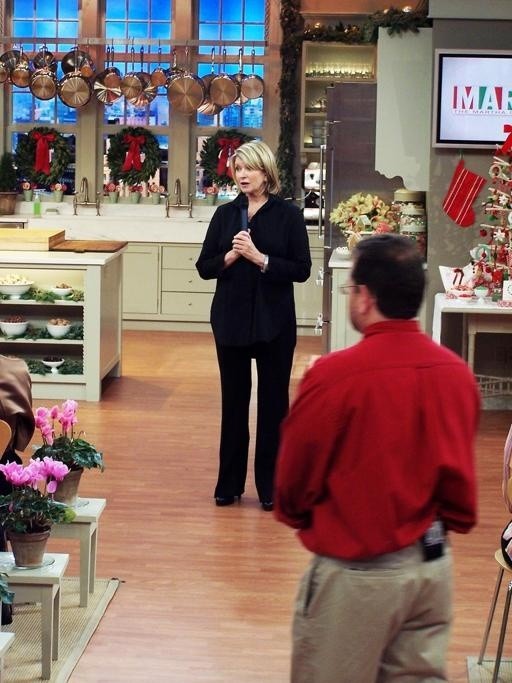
[318,80,405,357]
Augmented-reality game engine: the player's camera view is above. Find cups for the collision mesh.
[306,62,373,79]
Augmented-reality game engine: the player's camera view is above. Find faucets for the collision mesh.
[79,177,93,204]
[173,179,184,206]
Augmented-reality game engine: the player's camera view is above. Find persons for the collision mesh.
[274,233,481,681]
[195,139,311,512]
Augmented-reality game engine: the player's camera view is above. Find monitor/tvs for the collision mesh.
[429,48,512,150]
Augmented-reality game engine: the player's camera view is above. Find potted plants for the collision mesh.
[0,153,19,215]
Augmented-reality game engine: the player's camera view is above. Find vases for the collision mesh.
[47,467,83,502]
[7,526,51,567]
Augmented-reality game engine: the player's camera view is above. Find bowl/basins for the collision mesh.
[311,119,325,147]
[449,288,489,305]
[50,286,73,297]
[0,321,29,338]
[46,324,72,338]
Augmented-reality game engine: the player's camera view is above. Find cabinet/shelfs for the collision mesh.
[122,245,159,320]
[327,248,429,359]
[285,227,323,337]
[159,246,218,322]
[298,41,377,223]
[0,244,128,402]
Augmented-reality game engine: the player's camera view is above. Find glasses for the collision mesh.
[338,283,360,296]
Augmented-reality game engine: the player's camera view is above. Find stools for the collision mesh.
[0,552,69,680]
[477,549,512,683]
[0,632,15,683]
[29,498,106,607]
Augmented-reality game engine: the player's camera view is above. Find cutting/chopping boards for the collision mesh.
[50,240,128,252]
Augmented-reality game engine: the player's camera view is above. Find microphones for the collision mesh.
[233,190,250,236]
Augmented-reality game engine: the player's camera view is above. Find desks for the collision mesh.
[432,292,512,399]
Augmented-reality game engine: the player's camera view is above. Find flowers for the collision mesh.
[32,399,107,473]
[0,455,76,546]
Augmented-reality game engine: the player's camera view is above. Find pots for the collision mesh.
[0,44,265,116]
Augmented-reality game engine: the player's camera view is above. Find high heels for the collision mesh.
[259,495,274,512]
[216,495,242,506]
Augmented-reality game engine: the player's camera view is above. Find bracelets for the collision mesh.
[260,255,268,272]
